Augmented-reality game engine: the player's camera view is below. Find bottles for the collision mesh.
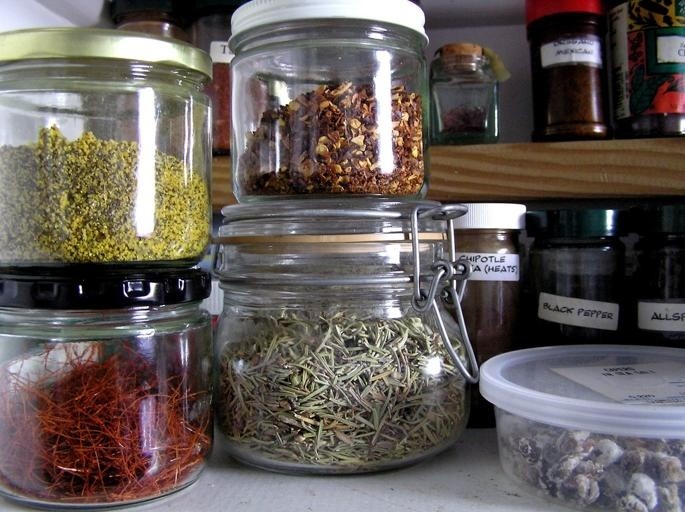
[117,19,232,157]
[436,38,502,137]
[0,26,218,275]
[1,265,219,511]
[443,200,529,428]
[221,198,479,474]
[478,340,685,511]
[529,203,644,344]
[225,0,435,204]
[629,196,685,345]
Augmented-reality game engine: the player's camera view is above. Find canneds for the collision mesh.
[447,203,684,429]
